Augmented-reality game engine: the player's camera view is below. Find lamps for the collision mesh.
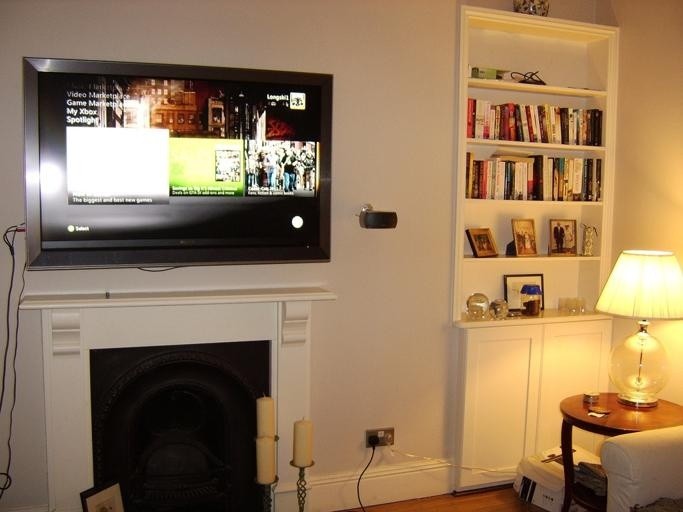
[593,247,682,411]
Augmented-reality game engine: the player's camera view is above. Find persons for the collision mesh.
[554,222,573,253]
[248,147,315,192]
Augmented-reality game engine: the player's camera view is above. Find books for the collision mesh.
[466,99,603,201]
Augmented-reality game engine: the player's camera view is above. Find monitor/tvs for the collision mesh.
[22,56,334,272]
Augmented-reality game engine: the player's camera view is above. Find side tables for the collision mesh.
[551,390,682,512]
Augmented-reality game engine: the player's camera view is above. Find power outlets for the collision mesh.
[365,427,395,447]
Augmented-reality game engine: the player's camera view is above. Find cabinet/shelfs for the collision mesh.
[457,7,621,496]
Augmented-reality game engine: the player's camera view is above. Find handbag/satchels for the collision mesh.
[574,462,607,496]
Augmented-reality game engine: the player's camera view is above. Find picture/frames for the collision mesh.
[504,274,545,312]
[466,218,578,257]
